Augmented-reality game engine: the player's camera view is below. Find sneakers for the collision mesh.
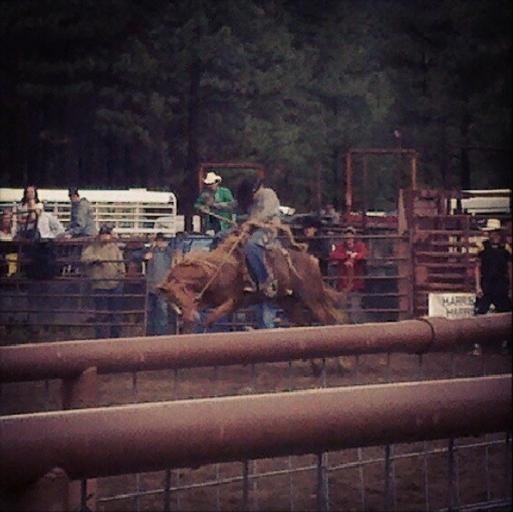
[243,279,263,293]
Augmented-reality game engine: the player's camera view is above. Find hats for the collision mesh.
[201,171,224,184]
[481,218,506,232]
[302,216,320,228]
[239,175,263,201]
[343,226,356,233]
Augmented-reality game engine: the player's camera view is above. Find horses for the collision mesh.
[154,244,355,377]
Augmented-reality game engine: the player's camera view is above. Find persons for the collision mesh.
[472,218,512,356]
[0,185,176,338]
[195,172,370,308]
